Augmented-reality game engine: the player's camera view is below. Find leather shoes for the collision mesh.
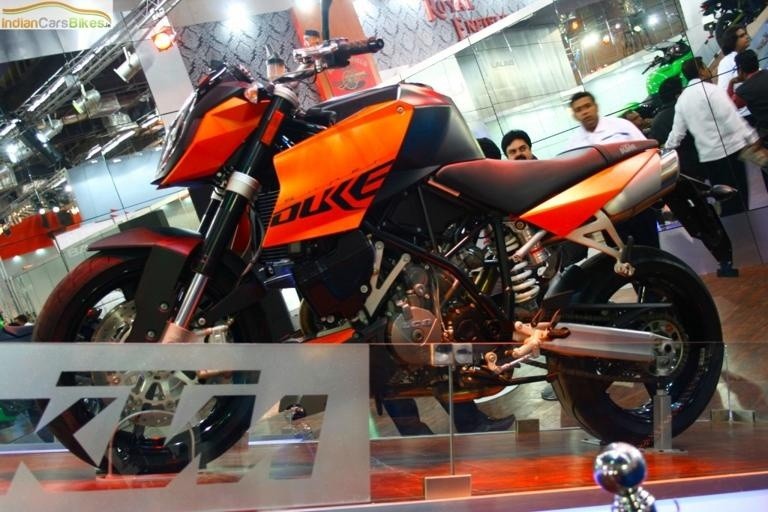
[472,414,515,431]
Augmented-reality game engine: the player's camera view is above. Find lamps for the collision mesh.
[33,50,143,145]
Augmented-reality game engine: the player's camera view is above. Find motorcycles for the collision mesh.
[27,0,724,478]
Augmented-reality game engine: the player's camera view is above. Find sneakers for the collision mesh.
[542,385,557,399]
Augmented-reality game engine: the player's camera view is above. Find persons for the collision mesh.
[564,93,659,299]
[477,129,538,161]
[614,24,768,220]
[9,308,36,341]
